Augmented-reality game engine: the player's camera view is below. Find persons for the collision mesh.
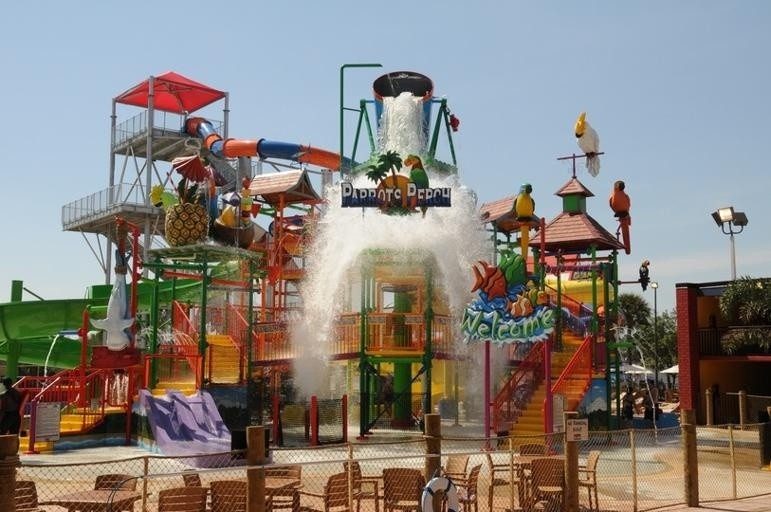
[641,379,660,429]
[102,369,129,407]
[383,372,394,418]
[665,383,673,403]
[621,386,640,427]
[0,377,22,453]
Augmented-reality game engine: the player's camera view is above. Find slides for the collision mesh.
[179,116,364,297]
[138,390,229,447]
[0,262,252,381]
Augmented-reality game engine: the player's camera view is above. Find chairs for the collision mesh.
[16,480,36,509]
[94,443,601,511]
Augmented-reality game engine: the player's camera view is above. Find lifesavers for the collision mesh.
[422,476,459,512]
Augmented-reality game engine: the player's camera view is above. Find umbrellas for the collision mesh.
[658,363,680,375]
[609,361,655,377]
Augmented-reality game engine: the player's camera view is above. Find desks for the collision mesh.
[38,489,152,511]
[512,455,566,507]
[208,477,301,512]
[421,473,467,511]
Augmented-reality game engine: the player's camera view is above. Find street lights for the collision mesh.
[713,206,750,283]
